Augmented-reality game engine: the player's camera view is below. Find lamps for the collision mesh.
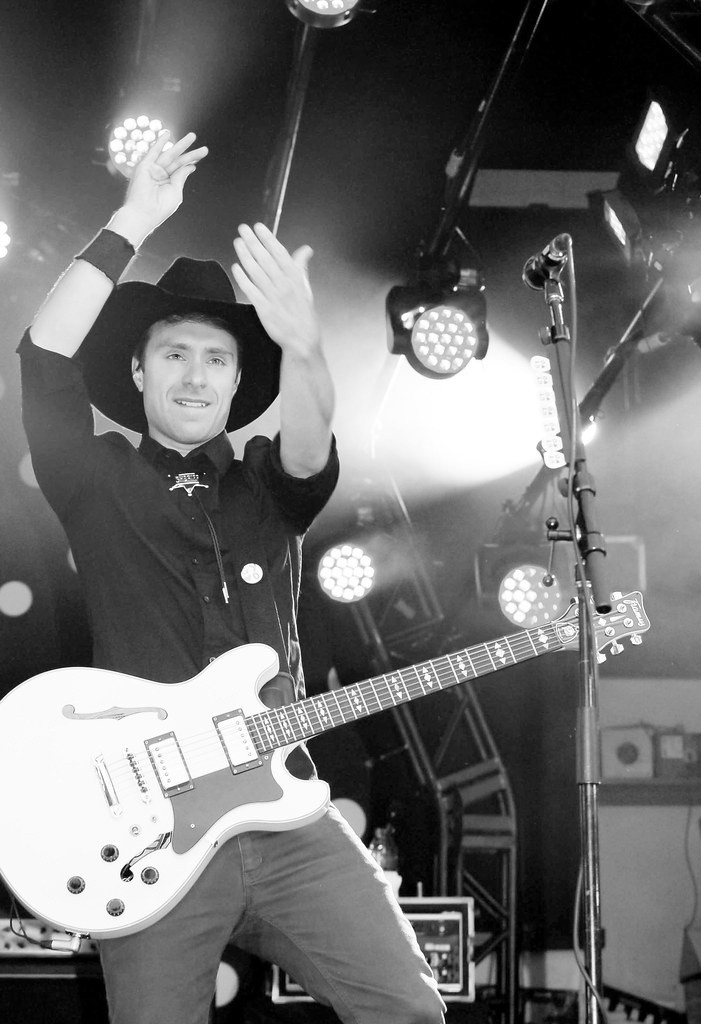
[285,0,363,28]
[475,502,571,630]
[587,97,700,289]
[107,65,180,181]
[385,0,552,381]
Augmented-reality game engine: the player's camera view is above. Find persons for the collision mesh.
[16,131,447,1024]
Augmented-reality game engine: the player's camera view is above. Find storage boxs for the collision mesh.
[272,897,474,1005]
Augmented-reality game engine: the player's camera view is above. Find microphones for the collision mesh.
[522,234,570,291]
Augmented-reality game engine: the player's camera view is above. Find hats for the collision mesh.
[74,257,282,435]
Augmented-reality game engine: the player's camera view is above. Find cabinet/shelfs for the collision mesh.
[592,679,700,1024]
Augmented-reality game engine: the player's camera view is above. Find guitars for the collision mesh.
[1,587,654,946]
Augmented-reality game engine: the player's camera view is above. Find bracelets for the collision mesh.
[74,229,136,286]
